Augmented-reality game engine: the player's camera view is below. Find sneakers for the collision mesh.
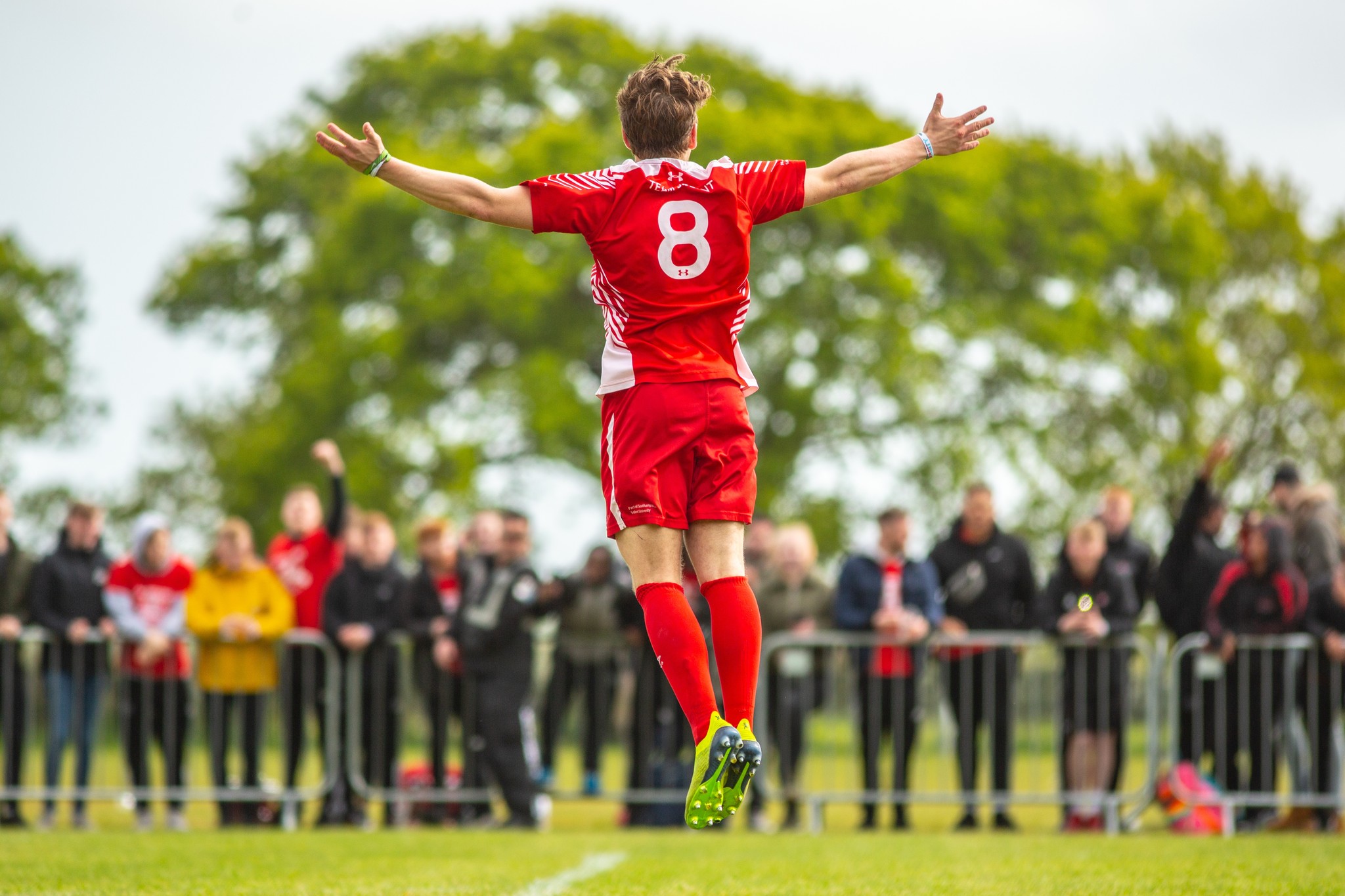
[712,718,762,824]
[684,709,744,830]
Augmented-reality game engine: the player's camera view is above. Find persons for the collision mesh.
[1,438,1344,834]
[317,56,995,832]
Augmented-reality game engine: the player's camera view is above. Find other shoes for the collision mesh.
[456,802,493,827]
[958,812,978,827]
[892,803,908,828]
[1269,806,1345,834]
[1061,811,1105,833]
[859,801,879,829]
[0,809,29,826]
[581,771,602,796]
[532,768,557,793]
[134,812,154,833]
[500,812,539,832]
[70,810,93,831]
[993,813,1014,830]
[39,809,56,829]
[164,811,190,831]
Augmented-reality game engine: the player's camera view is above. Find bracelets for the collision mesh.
[917,132,934,160]
[369,153,392,177]
[363,149,389,175]
